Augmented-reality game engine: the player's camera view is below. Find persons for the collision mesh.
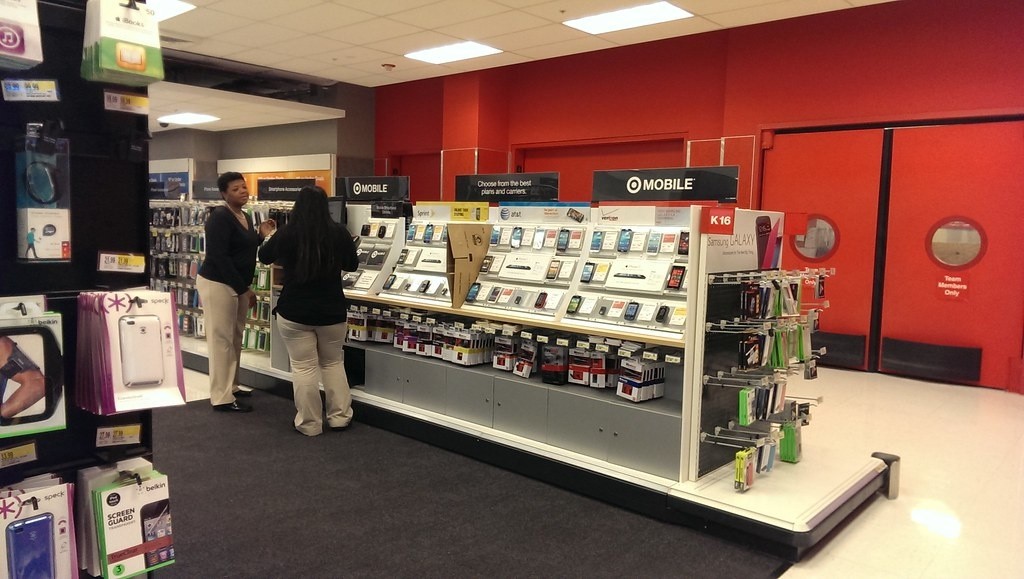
[196,171,277,413]
[0,335,46,419]
[258,186,360,437]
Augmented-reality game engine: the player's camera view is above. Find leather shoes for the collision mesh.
[233,389,251,397]
[293,419,313,437]
[329,423,347,430]
[214,400,253,413]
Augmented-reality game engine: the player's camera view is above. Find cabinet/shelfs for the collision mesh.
[365,344,683,481]
